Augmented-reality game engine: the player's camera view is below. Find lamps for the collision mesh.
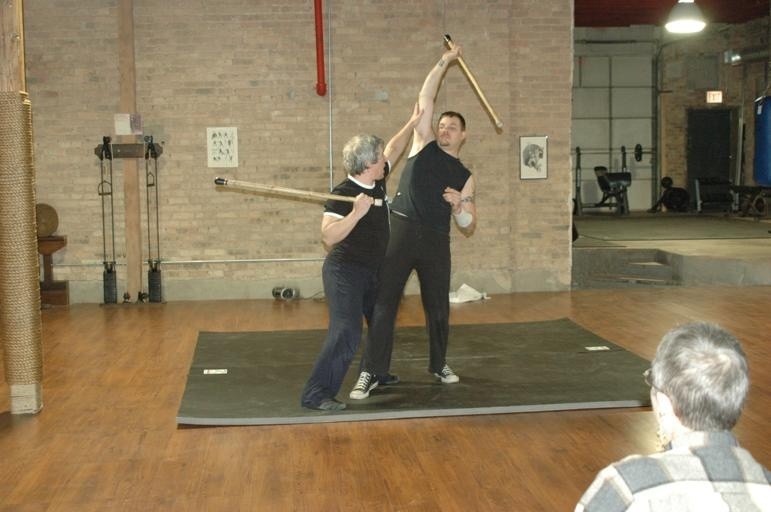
[664,1,708,36]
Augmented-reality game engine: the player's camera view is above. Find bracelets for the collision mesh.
[452,209,473,227]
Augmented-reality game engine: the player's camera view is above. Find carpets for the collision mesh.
[573,211,771,241]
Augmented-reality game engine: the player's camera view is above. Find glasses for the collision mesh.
[643,368,670,398]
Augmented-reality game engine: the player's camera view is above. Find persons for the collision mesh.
[350,46,476,404]
[299,102,423,412]
[573,318,771,511]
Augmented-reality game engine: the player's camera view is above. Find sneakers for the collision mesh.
[349,369,381,401]
[356,372,403,386]
[428,362,461,384]
[301,390,348,412]
